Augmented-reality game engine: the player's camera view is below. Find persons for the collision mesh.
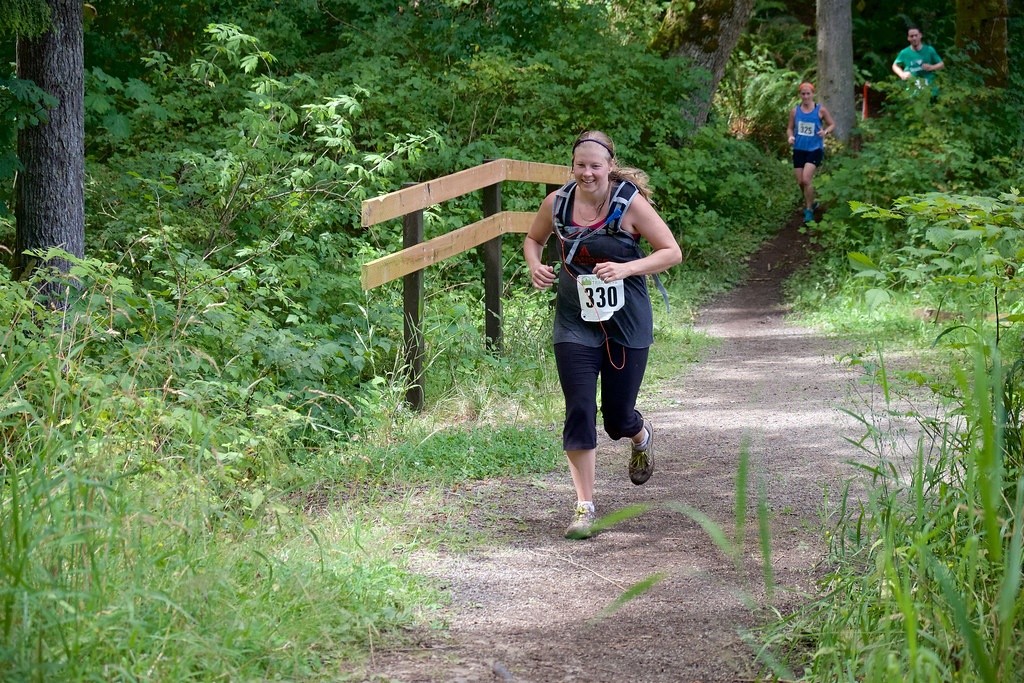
[523,132,682,539]
[891,26,945,105]
[786,81,837,223]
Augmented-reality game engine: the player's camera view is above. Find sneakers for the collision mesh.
[801,201,820,224]
[628,417,655,485]
[563,503,597,540]
